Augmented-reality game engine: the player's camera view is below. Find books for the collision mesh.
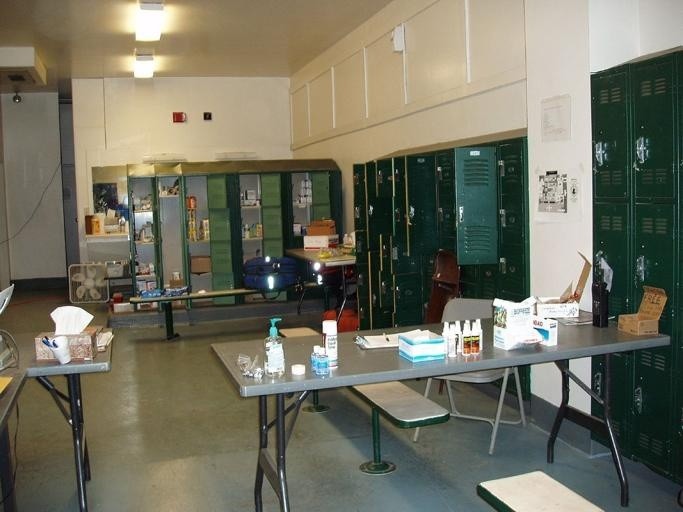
[98,331,112,347]
[361,329,424,349]
[106,333,114,346]
[97,345,106,352]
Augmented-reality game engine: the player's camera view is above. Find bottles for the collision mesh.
[442,318,484,361]
[310,344,331,377]
[320,320,339,370]
[589,270,611,328]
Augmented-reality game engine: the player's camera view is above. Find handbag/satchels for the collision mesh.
[243,256,297,291]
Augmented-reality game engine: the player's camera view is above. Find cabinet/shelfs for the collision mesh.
[589,57,682,481]
[351,137,532,403]
[122,156,343,313]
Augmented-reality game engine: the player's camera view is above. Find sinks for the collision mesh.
[104,208,118,231]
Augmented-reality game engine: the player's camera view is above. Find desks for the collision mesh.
[206,308,671,512]
[285,248,355,315]
[0,325,114,512]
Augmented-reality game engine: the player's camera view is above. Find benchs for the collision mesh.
[128,282,337,340]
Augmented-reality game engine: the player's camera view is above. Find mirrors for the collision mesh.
[90,165,127,220]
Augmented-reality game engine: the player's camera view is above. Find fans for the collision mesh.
[67,263,110,305]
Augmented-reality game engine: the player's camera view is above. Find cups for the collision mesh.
[49,336,72,365]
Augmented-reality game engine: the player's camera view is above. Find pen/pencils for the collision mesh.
[382,332,389,342]
[41,335,58,348]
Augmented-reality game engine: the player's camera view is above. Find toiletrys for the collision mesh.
[314,348,329,377]
[310,345,319,371]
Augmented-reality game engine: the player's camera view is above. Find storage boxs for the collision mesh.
[302,219,339,252]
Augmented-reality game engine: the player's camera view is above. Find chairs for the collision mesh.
[410,296,529,456]
